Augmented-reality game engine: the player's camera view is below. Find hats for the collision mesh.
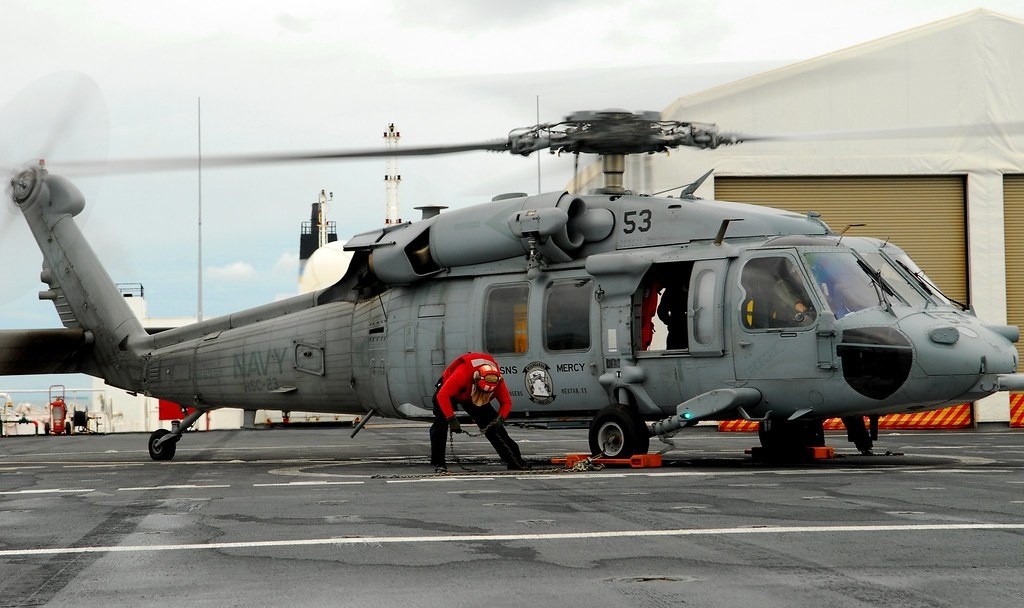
[473,365,503,393]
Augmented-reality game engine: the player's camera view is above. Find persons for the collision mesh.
[658,263,866,350]
[431,353,532,473]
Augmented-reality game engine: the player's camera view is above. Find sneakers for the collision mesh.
[507,459,532,470]
[434,466,447,472]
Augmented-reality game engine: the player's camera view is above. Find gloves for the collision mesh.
[449,416,461,433]
[493,416,504,428]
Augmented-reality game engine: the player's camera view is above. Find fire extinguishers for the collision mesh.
[51,396,66,435]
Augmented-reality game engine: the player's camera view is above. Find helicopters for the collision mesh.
[0,108,1024,469]
